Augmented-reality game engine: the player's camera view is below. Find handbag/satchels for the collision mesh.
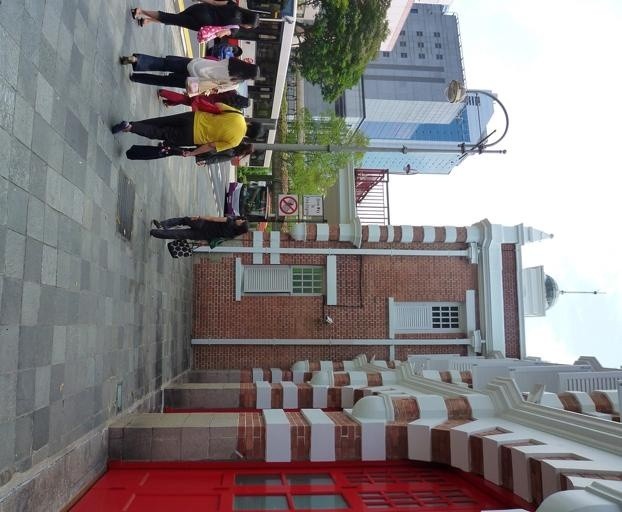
[125,142,185,160]
[167,239,200,259]
[196,24,241,45]
[191,95,224,115]
[206,238,227,250]
[183,75,220,99]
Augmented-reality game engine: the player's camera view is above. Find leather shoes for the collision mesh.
[153,219,162,229]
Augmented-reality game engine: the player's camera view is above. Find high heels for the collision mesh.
[138,17,145,27]
[111,120,131,134]
[131,7,140,20]
[119,55,134,65]
